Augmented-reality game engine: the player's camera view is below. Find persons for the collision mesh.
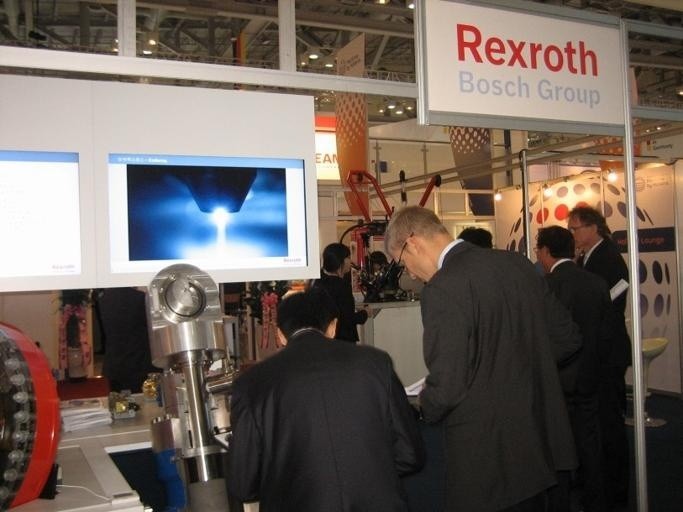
[566,207,633,511]
[383,205,582,511]
[363,210,612,303]
[96,289,153,393]
[317,242,373,342]
[535,226,630,512]
[227,293,427,512]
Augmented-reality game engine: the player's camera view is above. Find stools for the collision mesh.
[623,338,668,428]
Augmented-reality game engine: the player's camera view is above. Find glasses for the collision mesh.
[393,231,415,273]
[569,223,591,235]
